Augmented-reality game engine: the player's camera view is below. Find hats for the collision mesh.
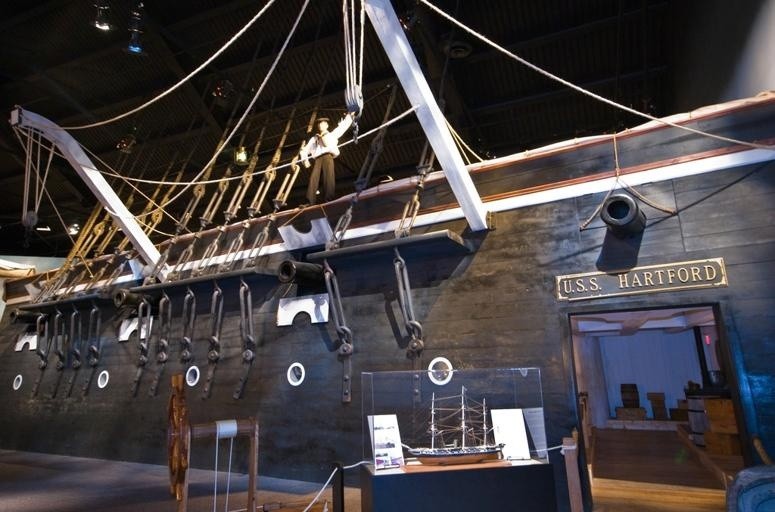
[315,117,330,122]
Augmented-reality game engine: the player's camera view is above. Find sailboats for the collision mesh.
[404,384,511,465]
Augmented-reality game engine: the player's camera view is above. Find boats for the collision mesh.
[0,0,775,512]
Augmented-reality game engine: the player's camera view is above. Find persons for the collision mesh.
[299,112,356,207]
